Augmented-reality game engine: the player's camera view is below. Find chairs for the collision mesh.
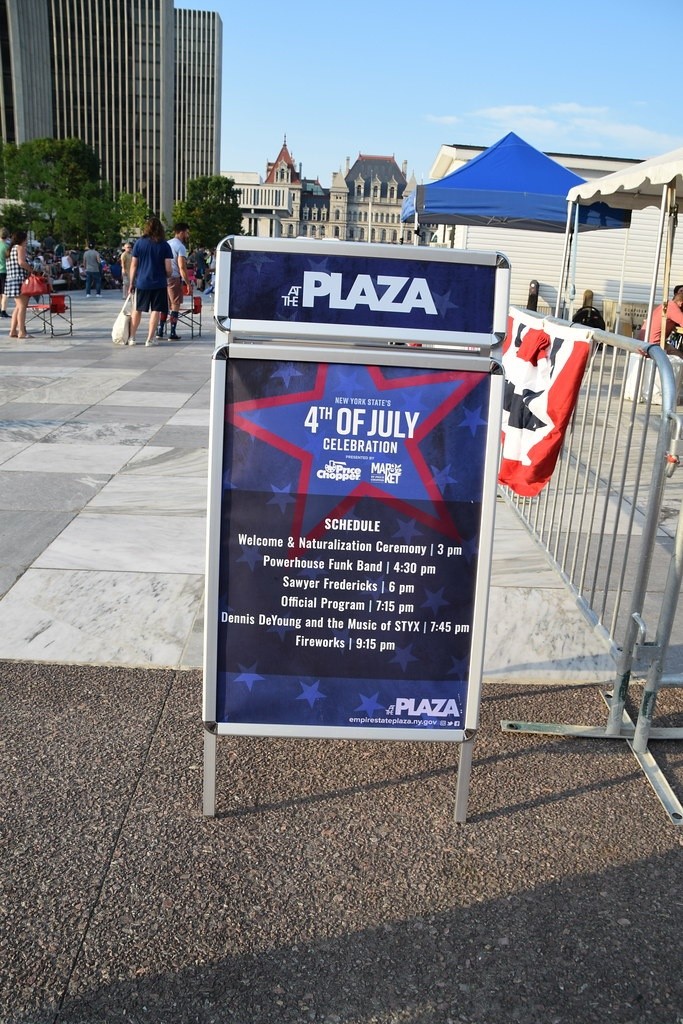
[23,294,74,339]
[156,283,203,339]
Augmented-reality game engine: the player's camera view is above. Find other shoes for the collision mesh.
[156,334,163,338]
[168,336,182,341]
[128,340,136,345]
[144,341,159,346]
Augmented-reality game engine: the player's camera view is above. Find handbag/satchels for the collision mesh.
[20,272,51,295]
[111,293,131,345]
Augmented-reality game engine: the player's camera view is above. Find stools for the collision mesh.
[624,353,683,406]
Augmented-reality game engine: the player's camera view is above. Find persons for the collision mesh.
[0,227,14,318]
[5,231,36,338]
[155,223,193,341]
[637,286,683,359]
[127,217,173,347]
[23,233,218,302]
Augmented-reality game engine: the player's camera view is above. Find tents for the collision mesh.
[396,132,633,338]
[553,145,683,324]
